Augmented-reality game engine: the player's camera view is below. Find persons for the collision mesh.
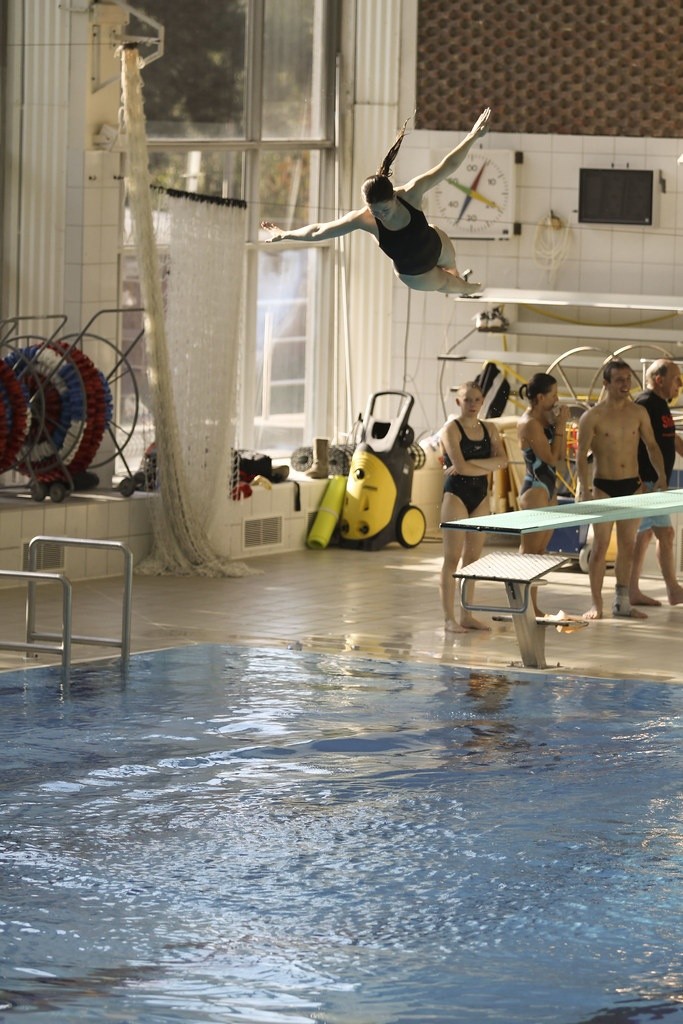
[628,358,683,608]
[438,382,506,633]
[262,106,492,293]
[515,373,571,615]
[574,360,667,620]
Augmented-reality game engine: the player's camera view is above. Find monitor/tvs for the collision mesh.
[579,168,653,226]
